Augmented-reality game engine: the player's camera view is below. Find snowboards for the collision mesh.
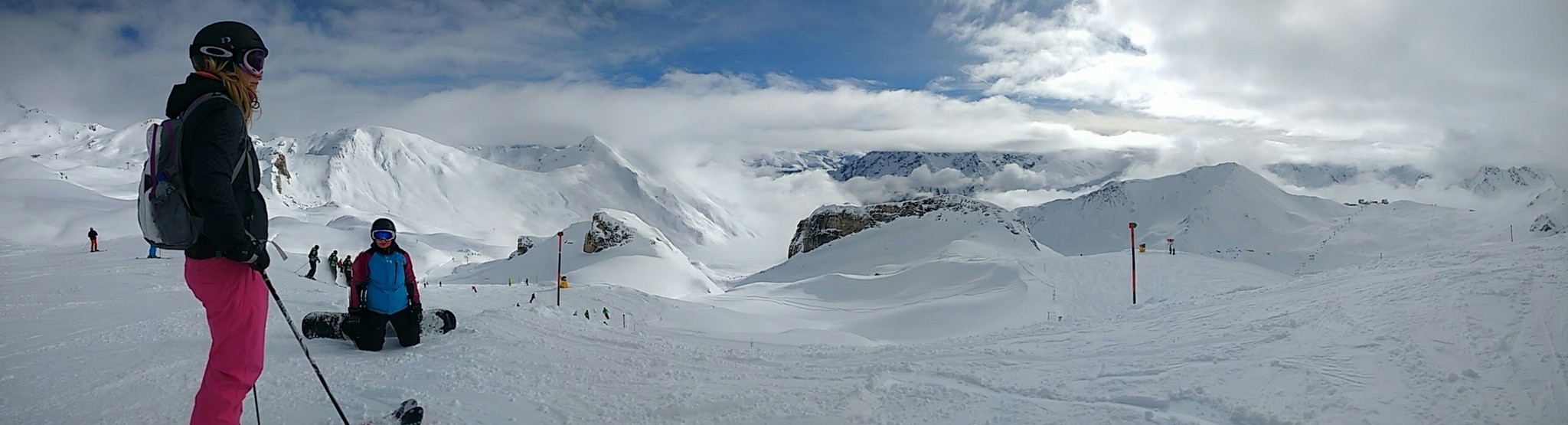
[301,308,457,340]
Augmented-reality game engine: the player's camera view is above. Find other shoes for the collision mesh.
[91,250,93,252]
[95,250,99,251]
[148,255,157,258]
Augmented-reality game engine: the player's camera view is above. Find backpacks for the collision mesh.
[137,93,253,251]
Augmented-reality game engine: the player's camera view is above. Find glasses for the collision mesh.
[372,231,395,240]
[238,48,265,76]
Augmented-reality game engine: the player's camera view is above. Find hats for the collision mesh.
[315,245,319,249]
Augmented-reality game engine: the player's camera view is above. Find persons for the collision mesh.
[526,278,529,286]
[1169,245,1175,255]
[166,21,269,425]
[148,245,161,259]
[424,281,442,288]
[306,245,354,287]
[584,310,590,320]
[472,286,477,292]
[529,293,536,304]
[348,218,423,352]
[602,307,609,320]
[88,227,100,252]
[508,278,511,286]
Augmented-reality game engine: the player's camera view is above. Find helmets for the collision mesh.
[90,227,93,229]
[371,219,396,240]
[189,21,268,76]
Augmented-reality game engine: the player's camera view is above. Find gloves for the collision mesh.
[348,307,361,329]
[317,259,320,263]
[412,302,423,323]
[242,241,269,270]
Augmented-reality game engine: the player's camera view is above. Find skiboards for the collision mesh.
[299,274,328,284]
[334,281,347,288]
[135,255,174,259]
[351,398,425,425]
[82,249,108,253]
